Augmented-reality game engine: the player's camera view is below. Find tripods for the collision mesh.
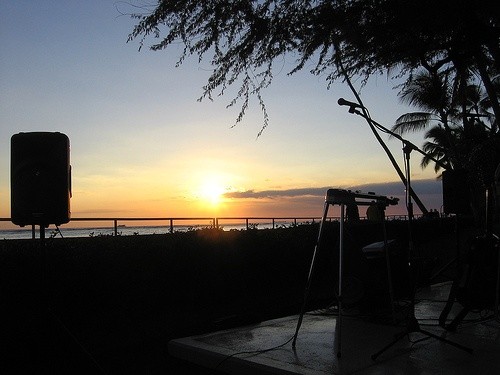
[348,109,476,361]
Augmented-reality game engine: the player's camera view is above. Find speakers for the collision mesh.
[441,169,469,216]
[9,132,72,226]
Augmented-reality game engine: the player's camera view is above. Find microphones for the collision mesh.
[338,98,366,109]
